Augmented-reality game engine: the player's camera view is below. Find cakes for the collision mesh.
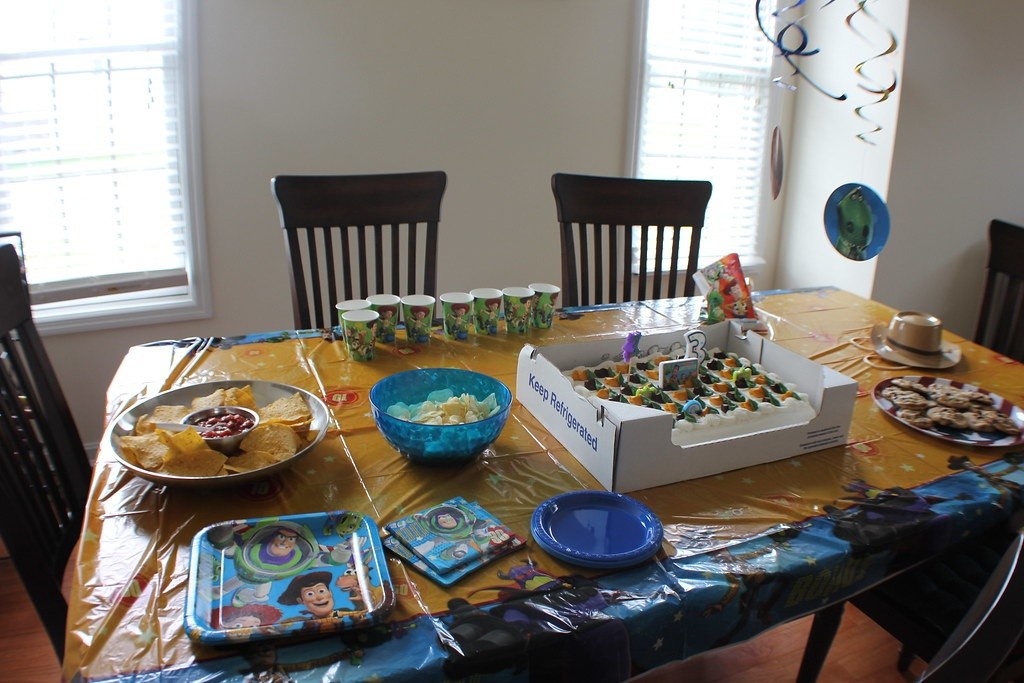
[562,346,816,446]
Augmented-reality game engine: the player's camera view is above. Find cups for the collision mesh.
[469,288,503,337]
[366,294,400,343]
[439,292,474,341]
[400,294,436,344]
[335,300,380,361]
[501,287,535,335]
[528,282,560,329]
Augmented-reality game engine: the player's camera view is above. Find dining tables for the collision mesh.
[58,284,1024,683]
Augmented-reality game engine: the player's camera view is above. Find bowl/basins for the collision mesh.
[369,368,514,466]
[179,405,260,453]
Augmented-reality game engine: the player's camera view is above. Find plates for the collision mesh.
[107,378,330,487]
[870,376,1024,447]
[183,509,396,648]
[530,490,664,570]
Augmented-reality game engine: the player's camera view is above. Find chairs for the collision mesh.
[550,172,713,308]
[0,243,94,668]
[911,528,1024,683]
[269,170,448,331]
[796,218,1024,683]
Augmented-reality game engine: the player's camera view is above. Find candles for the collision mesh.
[682,326,707,365]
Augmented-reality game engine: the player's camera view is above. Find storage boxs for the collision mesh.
[516,320,860,494]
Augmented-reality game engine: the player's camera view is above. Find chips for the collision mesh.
[387,388,500,426]
[119,384,316,477]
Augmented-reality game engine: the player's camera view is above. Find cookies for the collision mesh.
[881,377,1023,434]
